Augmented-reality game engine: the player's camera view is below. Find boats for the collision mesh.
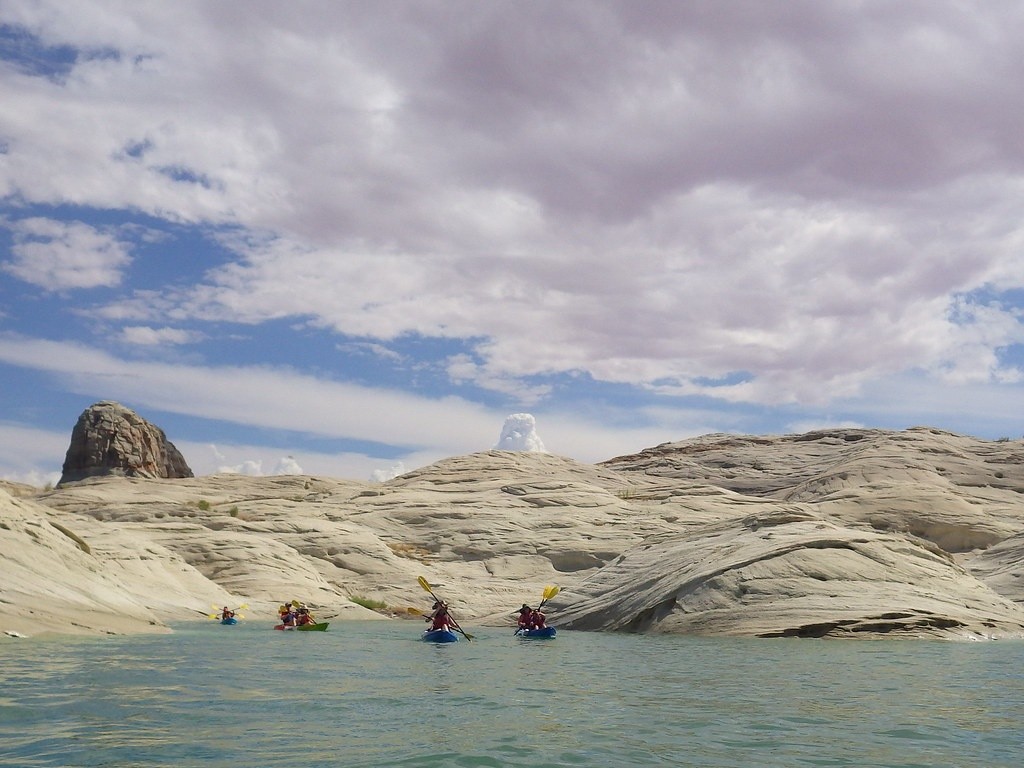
[219,617,237,625]
[421,629,459,643]
[274,622,330,631]
[515,626,557,639]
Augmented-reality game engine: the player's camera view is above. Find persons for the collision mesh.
[518,604,547,631]
[222,607,234,620]
[280,603,312,626]
[424,601,460,632]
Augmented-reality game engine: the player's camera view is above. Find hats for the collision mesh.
[285,603,292,608]
[520,604,532,614]
[441,601,448,607]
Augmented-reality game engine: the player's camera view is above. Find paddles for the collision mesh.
[278,600,317,624]
[208,603,247,621]
[513,585,560,636]
[407,576,476,641]
